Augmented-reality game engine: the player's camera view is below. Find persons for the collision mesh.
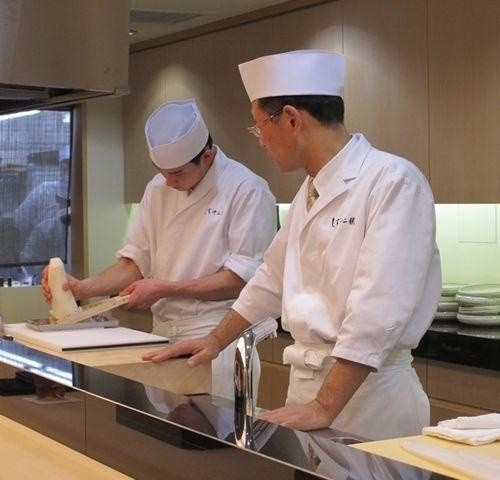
[41,98,277,407]
[18,187,71,284]
[142,50,442,440]
[14,145,70,231]
[145,386,234,441]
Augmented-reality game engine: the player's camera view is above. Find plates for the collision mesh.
[430,275,500,328]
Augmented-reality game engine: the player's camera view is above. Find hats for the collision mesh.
[144,97,209,171]
[237,48,347,102]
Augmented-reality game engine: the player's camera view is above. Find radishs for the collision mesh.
[48,258,79,320]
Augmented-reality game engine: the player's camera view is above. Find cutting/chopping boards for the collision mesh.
[346,434,500,479]
[4,321,171,351]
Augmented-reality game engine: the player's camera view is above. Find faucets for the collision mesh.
[232,315,279,454]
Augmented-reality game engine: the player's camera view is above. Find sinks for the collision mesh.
[330,436,363,446]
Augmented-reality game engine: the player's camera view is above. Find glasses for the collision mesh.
[246,107,283,138]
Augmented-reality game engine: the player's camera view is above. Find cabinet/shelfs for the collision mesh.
[121,37,165,203]
[165,21,216,145]
[428,1,500,204]
[344,0,428,181]
[217,0,343,203]
